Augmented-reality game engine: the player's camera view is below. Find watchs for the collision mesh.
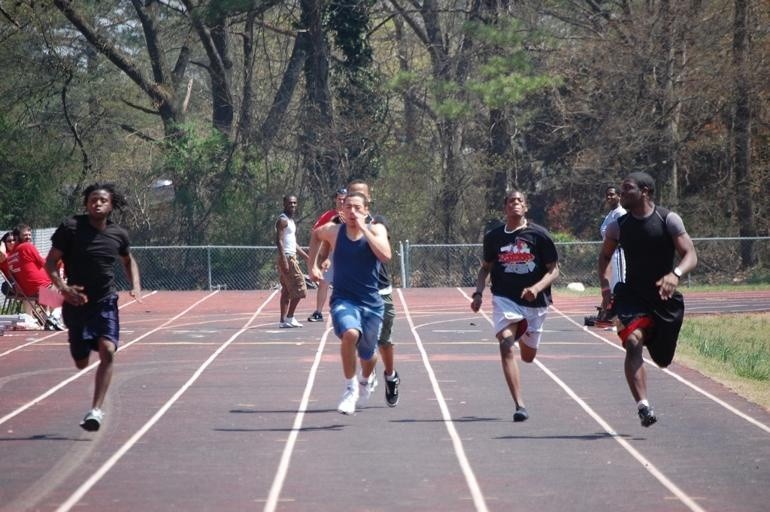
[673,268,684,279]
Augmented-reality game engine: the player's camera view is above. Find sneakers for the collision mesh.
[308,310,323,322]
[637,400,657,428]
[287,316,304,327]
[47,316,65,331]
[359,369,377,399]
[78,410,104,432]
[337,385,361,414]
[279,321,292,328]
[514,406,529,421]
[383,369,400,408]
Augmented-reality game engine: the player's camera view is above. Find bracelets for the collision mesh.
[602,288,610,293]
[471,292,482,298]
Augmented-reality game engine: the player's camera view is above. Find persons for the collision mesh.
[7,223,65,331]
[0,231,35,316]
[471,191,560,421]
[308,189,347,322]
[308,193,392,414]
[45,182,140,431]
[595,185,626,321]
[597,172,697,427]
[274,195,310,328]
[321,179,401,408]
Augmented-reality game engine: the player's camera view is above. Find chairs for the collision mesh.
[0,250,50,330]
